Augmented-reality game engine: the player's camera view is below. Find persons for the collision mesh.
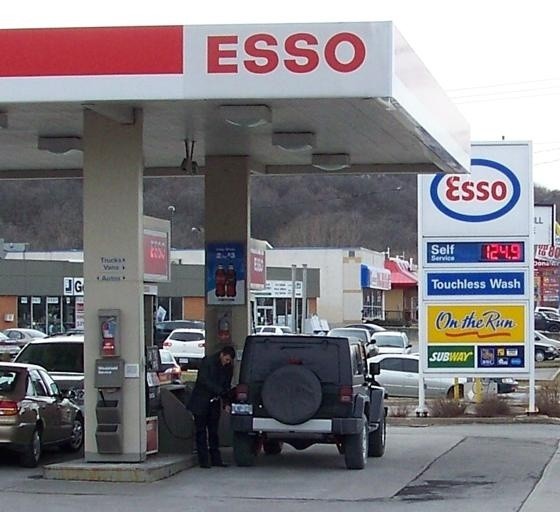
[186,345,237,468]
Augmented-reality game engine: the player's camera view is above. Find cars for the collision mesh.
[0,318,207,470]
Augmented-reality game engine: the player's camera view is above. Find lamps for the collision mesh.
[37,137,84,157]
[270,132,318,153]
[310,154,351,172]
[217,104,273,129]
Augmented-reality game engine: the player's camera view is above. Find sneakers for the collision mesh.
[199,463,211,469]
[211,461,232,467]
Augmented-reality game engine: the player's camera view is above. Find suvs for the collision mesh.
[224,330,392,472]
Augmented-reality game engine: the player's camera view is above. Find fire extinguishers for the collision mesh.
[101,316,115,354]
[217,313,231,341]
